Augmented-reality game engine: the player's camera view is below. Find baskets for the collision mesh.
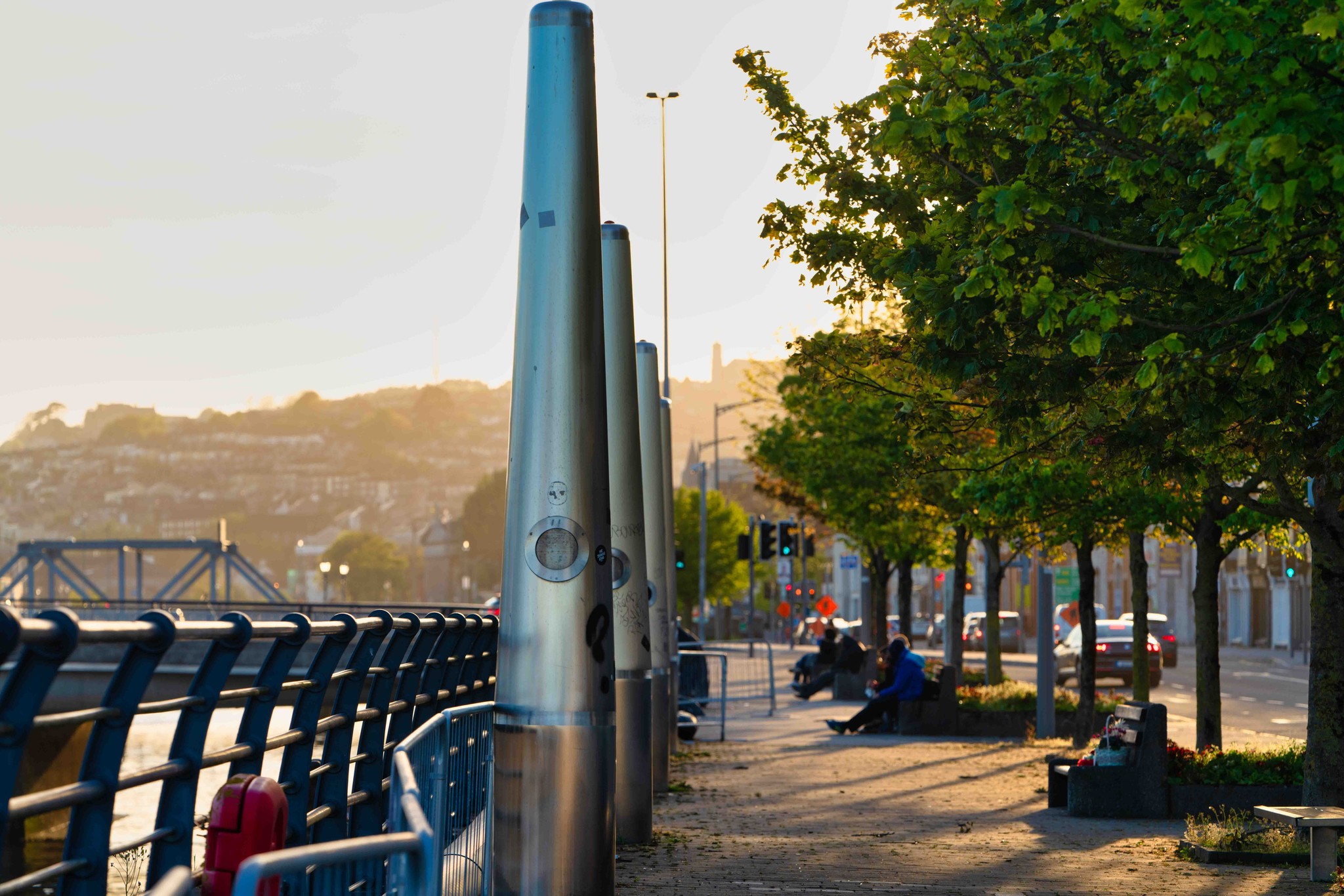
[1095,714,1131,767]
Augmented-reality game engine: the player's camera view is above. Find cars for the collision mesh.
[881,609,1027,654]
[1053,619,1163,688]
[795,617,854,645]
[1118,613,1177,667]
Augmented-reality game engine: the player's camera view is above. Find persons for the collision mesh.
[788,628,865,701]
[825,634,926,734]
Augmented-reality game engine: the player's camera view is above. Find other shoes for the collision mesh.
[792,682,811,700]
[825,719,859,734]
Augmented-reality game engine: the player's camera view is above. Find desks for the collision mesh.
[1254,805,1344,882]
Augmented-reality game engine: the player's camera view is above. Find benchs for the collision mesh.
[878,658,957,737]
[834,648,877,699]
[1045,698,1167,822]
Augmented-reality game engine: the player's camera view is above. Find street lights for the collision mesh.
[647,91,678,403]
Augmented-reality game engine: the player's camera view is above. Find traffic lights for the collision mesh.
[738,534,750,560]
[760,521,776,560]
[1287,543,1297,577]
[964,576,976,596]
[779,521,794,557]
[674,549,684,570]
[804,533,815,556]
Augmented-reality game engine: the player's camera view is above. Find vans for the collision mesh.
[1051,603,1109,645]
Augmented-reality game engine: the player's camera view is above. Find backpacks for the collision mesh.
[843,635,866,660]
